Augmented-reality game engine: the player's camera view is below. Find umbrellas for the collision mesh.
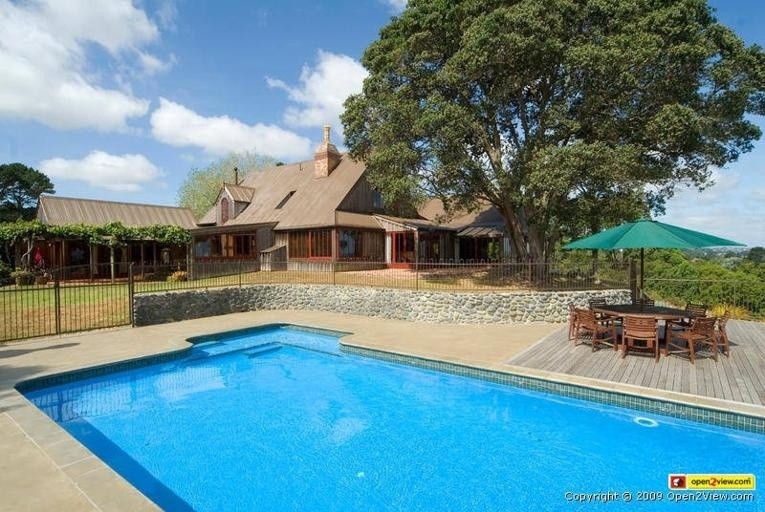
[559,218,750,310]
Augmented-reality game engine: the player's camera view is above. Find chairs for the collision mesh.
[569,297,731,364]
